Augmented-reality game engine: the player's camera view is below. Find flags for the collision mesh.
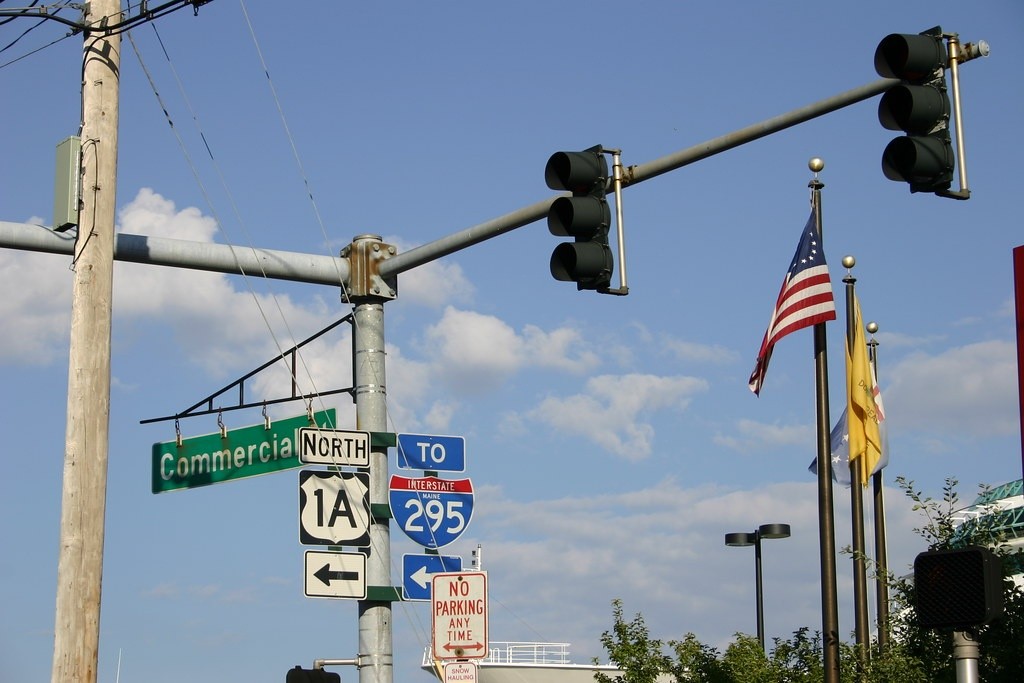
[749,211,889,490]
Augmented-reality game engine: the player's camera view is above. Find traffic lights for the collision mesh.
[915,545,1000,634]
[546,142,615,294]
[874,26,960,201]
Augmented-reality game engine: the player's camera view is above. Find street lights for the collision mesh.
[725,522,792,662]
